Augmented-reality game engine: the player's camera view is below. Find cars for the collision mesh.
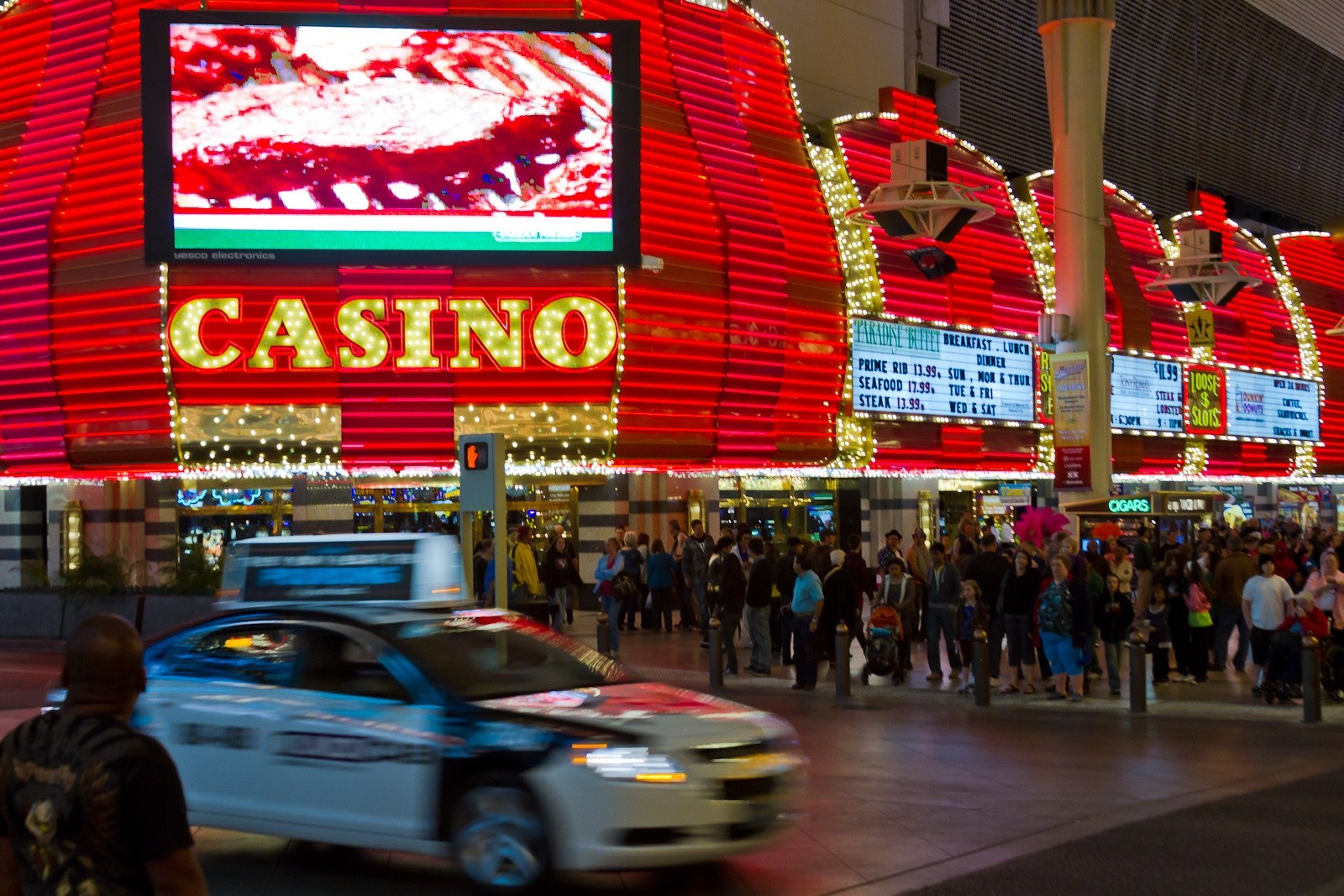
[44,601,806,894]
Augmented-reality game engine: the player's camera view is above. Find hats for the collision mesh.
[788,537,805,547]
[884,529,903,539]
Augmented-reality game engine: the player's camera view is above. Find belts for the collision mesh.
[795,611,815,618]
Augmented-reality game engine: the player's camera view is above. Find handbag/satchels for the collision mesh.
[611,571,639,600]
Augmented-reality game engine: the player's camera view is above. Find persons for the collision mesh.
[0,611,210,896]
[443,500,1339,713]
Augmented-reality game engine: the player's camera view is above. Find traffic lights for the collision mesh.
[464,441,489,472]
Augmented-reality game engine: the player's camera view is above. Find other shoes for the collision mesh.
[789,639,1249,702]
[554,611,771,679]
